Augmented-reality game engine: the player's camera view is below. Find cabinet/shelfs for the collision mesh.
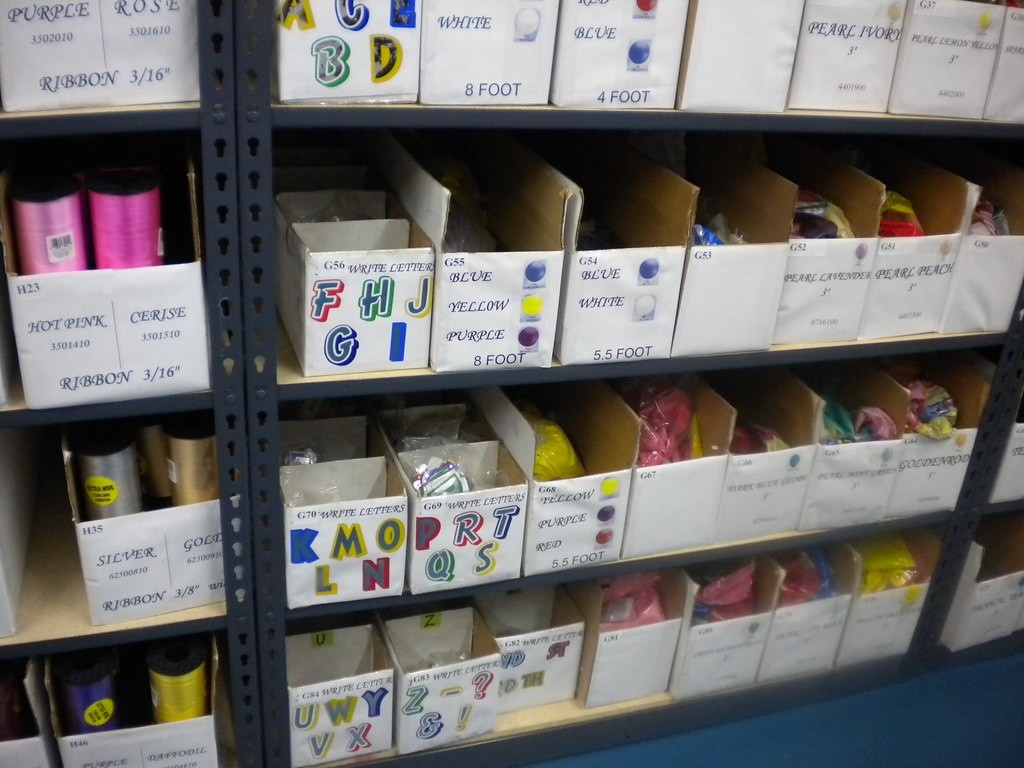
[0,1,267,768]
[916,332,1024,674]
[234,1,1024,768]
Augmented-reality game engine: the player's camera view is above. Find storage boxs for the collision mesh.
[0,1,1024,768]
